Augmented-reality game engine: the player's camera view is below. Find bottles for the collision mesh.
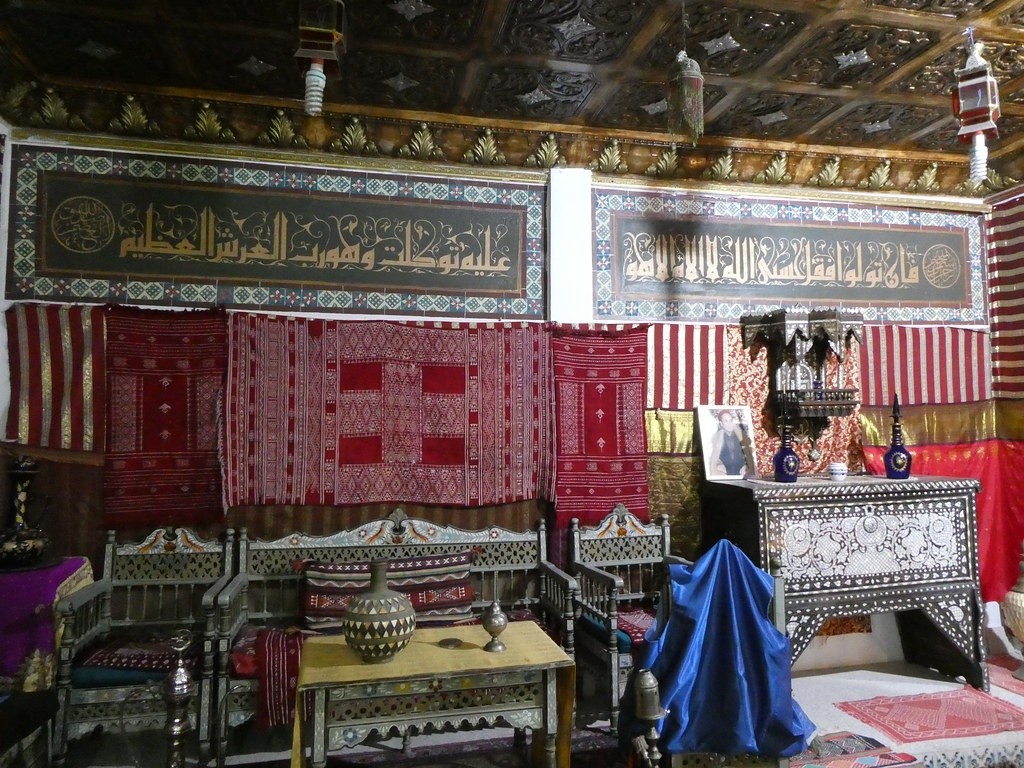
[883,423,912,478]
[0,469,51,567]
[773,425,800,483]
[342,559,416,664]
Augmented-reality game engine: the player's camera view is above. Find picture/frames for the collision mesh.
[697,404,762,481]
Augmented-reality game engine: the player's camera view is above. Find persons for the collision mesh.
[708,410,751,476]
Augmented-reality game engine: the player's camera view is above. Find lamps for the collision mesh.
[952,27,1002,182]
[668,56,704,151]
[296,0,348,117]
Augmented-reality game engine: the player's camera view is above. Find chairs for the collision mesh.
[53,526,235,768]
[566,503,695,737]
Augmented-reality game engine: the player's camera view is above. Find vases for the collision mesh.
[0,455,58,571]
[342,560,416,664]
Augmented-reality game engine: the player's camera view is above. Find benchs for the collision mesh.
[216,508,579,768]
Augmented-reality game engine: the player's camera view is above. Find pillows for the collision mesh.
[293,546,484,627]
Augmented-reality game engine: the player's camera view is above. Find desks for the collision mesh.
[285,619,578,768]
[0,554,94,768]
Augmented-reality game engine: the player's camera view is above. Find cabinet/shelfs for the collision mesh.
[703,469,992,694]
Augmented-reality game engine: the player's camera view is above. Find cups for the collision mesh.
[826,463,848,481]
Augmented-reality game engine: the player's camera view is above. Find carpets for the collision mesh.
[224,725,630,768]
[787,730,916,768]
[833,684,1024,746]
[985,652,1024,697]
